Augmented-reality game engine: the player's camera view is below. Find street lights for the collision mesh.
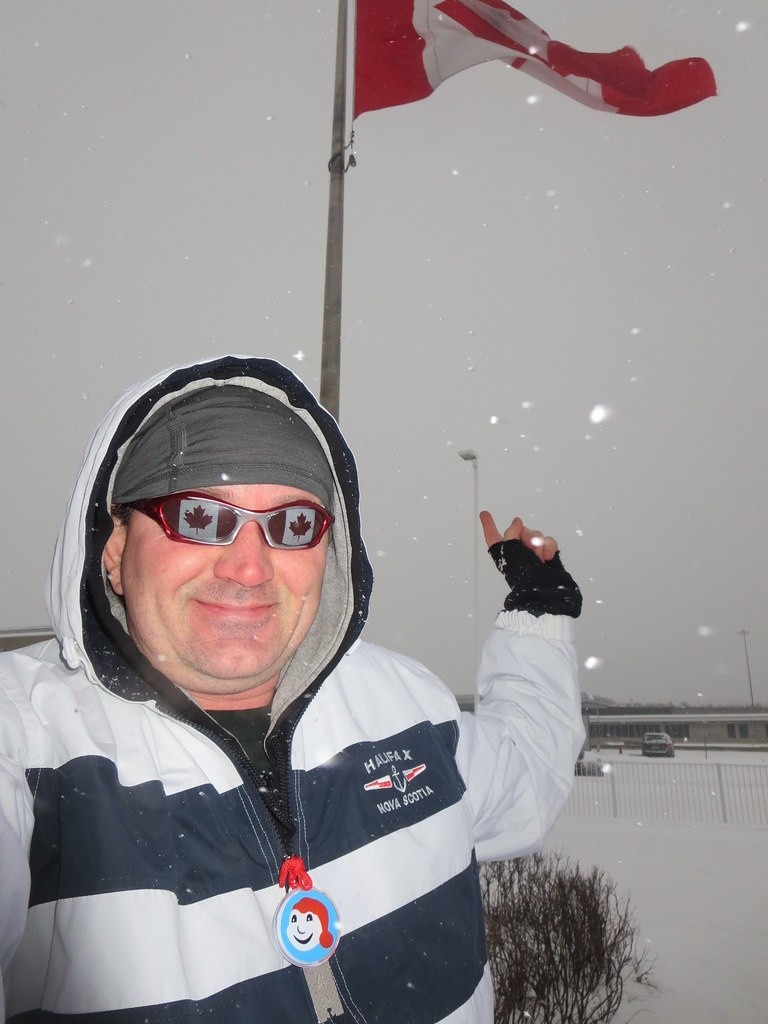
[460,446,484,709]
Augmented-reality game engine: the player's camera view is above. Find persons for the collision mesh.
[0,356,588,1024]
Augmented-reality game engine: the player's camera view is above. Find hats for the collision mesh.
[111,387,334,513]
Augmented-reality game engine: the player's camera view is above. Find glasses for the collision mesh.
[124,490,336,550]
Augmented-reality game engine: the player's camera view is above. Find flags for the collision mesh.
[328,0,718,173]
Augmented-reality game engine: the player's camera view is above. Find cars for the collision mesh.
[642,731,675,758]
